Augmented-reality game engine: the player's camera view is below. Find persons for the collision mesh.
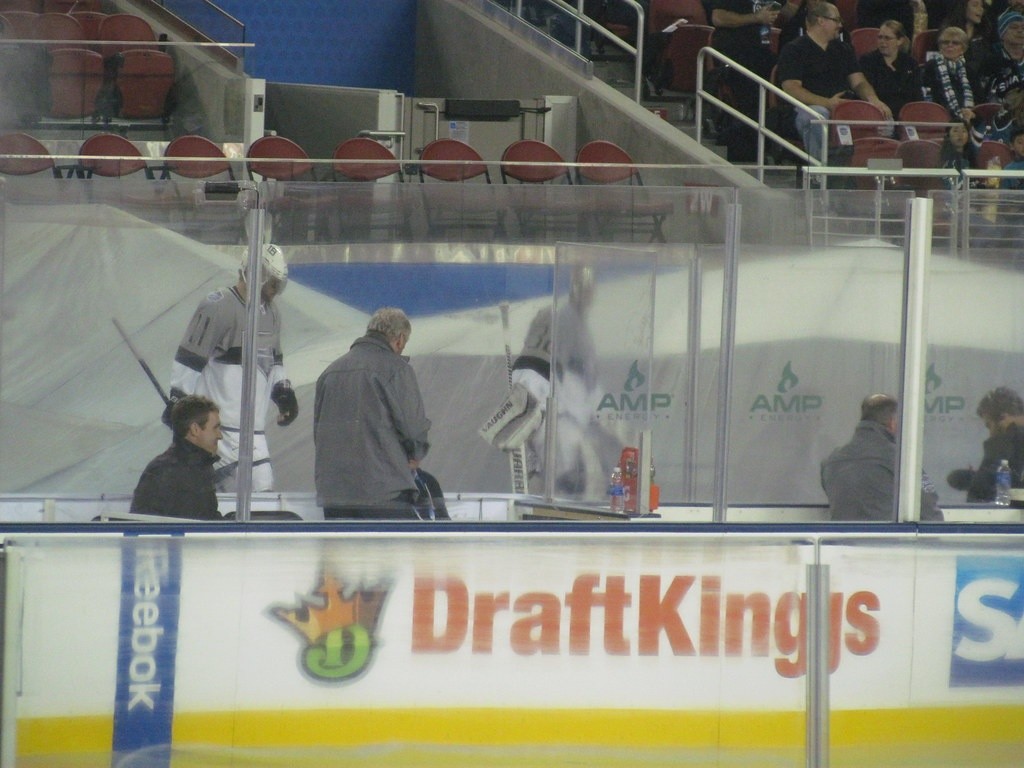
[702,0,1024,245]
[821,394,945,519]
[129,398,224,520]
[163,242,298,492]
[484,271,628,501]
[946,387,1024,504]
[314,308,448,522]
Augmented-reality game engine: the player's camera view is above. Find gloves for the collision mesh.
[983,422,1016,463]
[947,469,975,490]
[270,380,298,426]
[162,386,188,428]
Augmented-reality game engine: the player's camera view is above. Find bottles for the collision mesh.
[609,466,625,514]
[994,459,1012,506]
[983,126,993,141]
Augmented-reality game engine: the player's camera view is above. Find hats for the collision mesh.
[997,11,1024,39]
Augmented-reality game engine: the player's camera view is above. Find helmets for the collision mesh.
[237,244,289,287]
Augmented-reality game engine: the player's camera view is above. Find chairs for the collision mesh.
[0,0,1024,243]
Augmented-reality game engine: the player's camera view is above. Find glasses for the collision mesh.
[814,14,845,25]
[941,40,964,47]
[878,35,900,42]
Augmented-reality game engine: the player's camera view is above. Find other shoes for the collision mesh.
[702,113,721,139]
[810,173,831,189]
